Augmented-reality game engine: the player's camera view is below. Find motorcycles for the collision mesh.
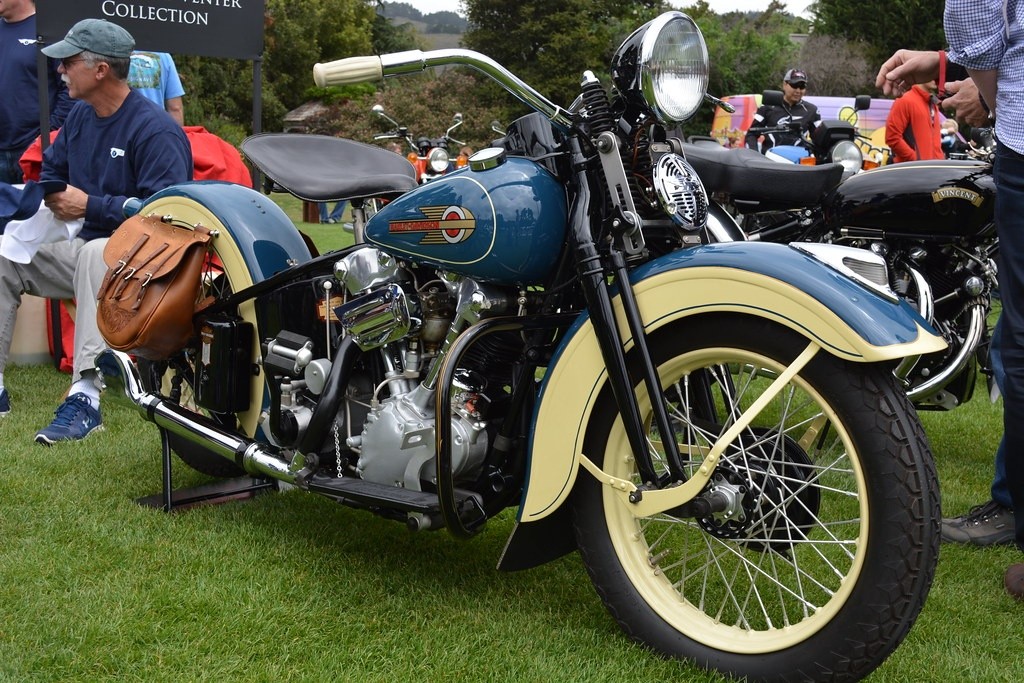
[104,7,957,683]
[345,89,1007,412]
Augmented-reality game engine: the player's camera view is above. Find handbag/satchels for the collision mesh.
[96,213,216,361]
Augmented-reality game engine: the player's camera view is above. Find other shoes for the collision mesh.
[1004,563,1024,601]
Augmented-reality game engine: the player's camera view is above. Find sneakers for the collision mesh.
[941,499,1015,545]
[0,389,12,418]
[34,392,105,447]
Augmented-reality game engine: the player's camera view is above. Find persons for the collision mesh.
[1,0,79,186]
[886,80,946,164]
[744,69,821,156]
[875,49,1016,547]
[455,147,474,170]
[317,201,347,224]
[127,50,184,126]
[386,142,402,156]
[943,0,1024,600]
[1,19,193,448]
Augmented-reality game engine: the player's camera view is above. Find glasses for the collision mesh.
[787,82,805,90]
[60,58,86,69]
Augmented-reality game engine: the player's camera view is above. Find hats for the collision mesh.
[784,68,808,84]
[41,18,136,58]
